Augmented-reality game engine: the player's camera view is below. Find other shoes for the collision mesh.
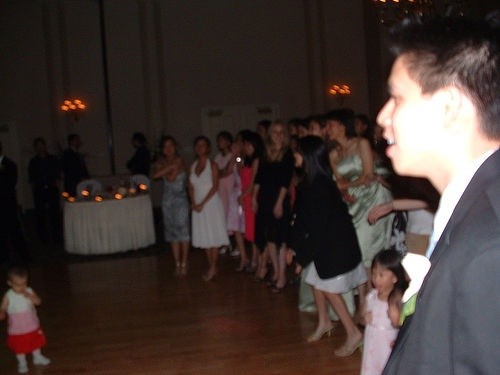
[33,357,51,365]
[17,362,28,373]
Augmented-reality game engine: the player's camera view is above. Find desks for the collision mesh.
[63,193,157,256]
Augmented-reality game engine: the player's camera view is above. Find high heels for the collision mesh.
[336,334,363,356]
[308,325,335,341]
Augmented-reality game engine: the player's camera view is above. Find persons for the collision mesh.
[124,108,440,355]
[386,279,409,327]
[28,135,93,246]
[376,18,500,375]
[0,132,29,260]
[360,249,405,375]
[0,265,52,373]
